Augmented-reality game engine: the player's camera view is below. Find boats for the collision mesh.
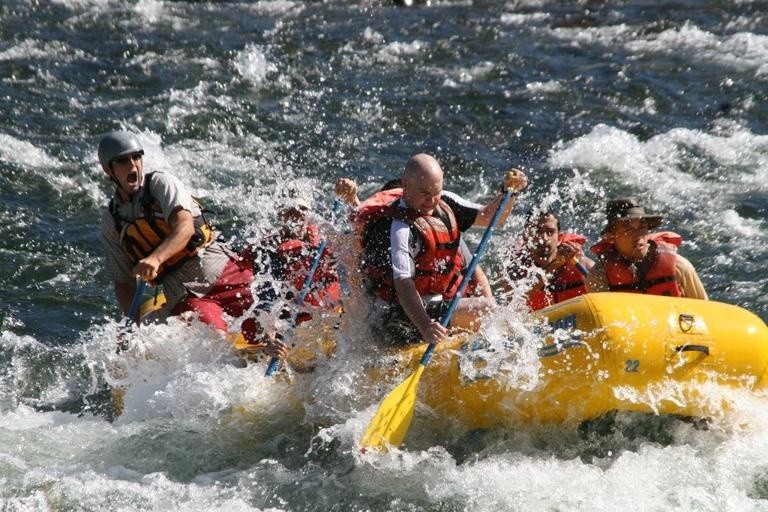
[102,319,339,424]
[299,290,767,436]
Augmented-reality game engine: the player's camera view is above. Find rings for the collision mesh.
[430,326,438,334]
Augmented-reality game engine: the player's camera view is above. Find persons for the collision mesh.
[97,130,260,334]
[587,199,710,302]
[504,208,593,312]
[241,179,359,387]
[364,153,528,355]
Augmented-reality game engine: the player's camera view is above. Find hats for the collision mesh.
[600,205,664,236]
[273,181,312,210]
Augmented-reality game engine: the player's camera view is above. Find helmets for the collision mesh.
[98,131,144,165]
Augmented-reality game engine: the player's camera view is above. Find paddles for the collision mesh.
[361,176,518,452]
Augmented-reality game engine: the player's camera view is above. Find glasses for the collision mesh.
[111,154,141,164]
